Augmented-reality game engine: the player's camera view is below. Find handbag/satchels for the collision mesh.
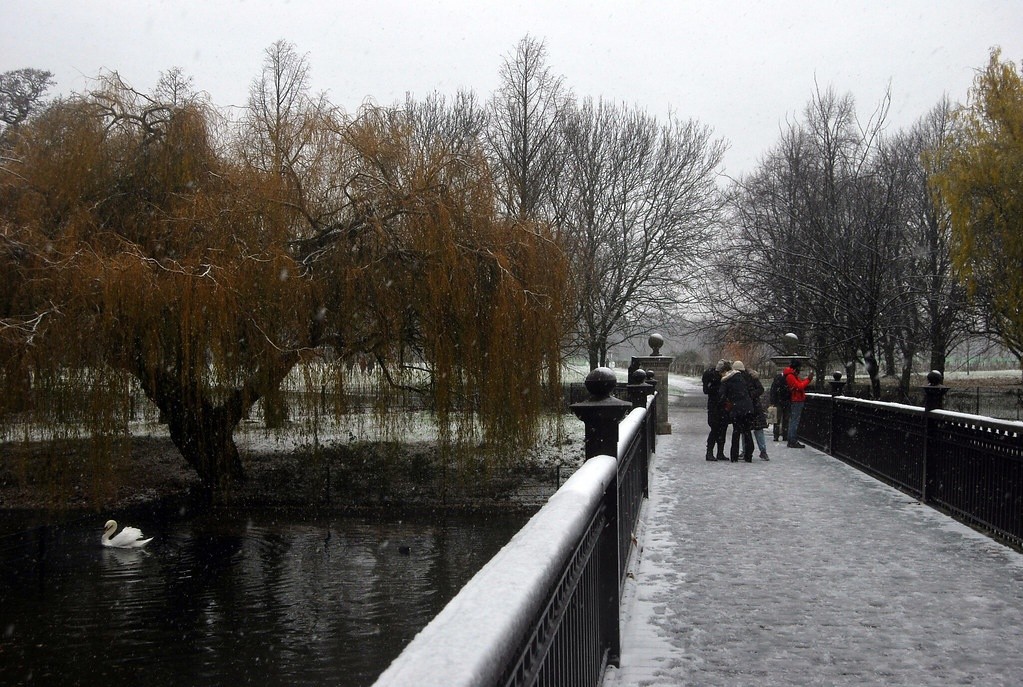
[724,399,733,413]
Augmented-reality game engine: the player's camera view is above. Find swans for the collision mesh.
[100,519,154,549]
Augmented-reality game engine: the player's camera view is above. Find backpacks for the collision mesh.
[770,372,797,409]
[703,367,722,395]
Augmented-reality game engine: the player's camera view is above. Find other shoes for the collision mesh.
[738,452,745,460]
[787,441,806,449]
[773,436,779,441]
[717,452,729,461]
[706,454,717,462]
[743,454,753,463]
[782,436,787,441]
[731,453,739,462]
[759,452,769,461]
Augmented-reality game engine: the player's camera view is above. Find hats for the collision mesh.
[716,359,725,371]
[732,360,745,371]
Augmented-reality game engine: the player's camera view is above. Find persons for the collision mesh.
[771,359,815,448]
[702,360,770,462]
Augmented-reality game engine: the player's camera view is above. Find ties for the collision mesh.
[789,358,803,369]
